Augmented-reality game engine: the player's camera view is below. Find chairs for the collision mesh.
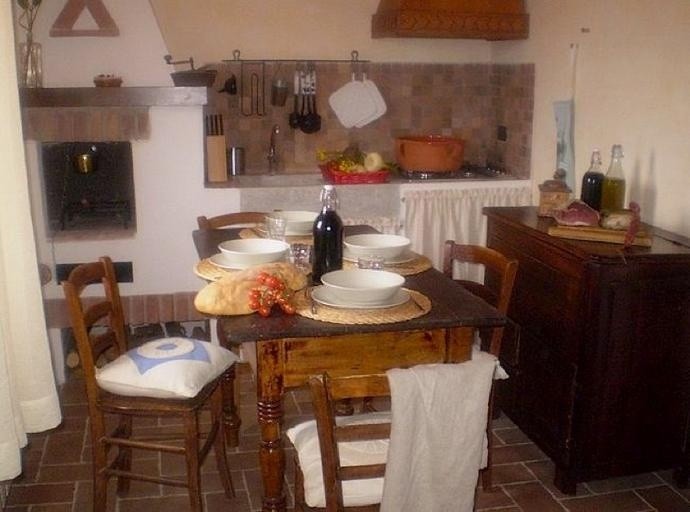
[61,255,238,512]
[360,240,518,494]
[197,209,282,229]
[294,350,499,511]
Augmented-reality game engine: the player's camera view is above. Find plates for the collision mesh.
[265,229,316,236]
[208,253,297,272]
[341,249,416,265]
[311,287,411,310]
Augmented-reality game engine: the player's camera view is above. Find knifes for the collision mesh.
[205,113,224,136]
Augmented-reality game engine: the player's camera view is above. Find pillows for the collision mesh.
[285,410,392,508]
[94,337,241,400]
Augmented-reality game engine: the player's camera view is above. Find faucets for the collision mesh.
[264,119,280,176]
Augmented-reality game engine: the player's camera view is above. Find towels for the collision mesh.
[379,351,510,511]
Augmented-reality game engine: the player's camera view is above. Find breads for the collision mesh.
[194,261,307,316]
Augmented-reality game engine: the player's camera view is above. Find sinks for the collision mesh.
[249,170,321,188]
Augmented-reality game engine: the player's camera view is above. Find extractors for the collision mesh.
[371,0,529,41]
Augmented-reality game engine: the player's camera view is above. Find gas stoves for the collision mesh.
[404,167,514,180]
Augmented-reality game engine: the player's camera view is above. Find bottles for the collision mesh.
[602,142,626,211]
[311,185,343,283]
[581,148,605,211]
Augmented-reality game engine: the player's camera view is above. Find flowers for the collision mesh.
[18,1,44,78]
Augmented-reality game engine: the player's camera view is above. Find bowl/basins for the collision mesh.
[266,211,319,232]
[395,133,466,173]
[321,268,405,304]
[207,212,267,242]
[218,238,290,266]
[345,234,411,260]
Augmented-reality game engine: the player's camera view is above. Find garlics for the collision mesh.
[365,152,384,173]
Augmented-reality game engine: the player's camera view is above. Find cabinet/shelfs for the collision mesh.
[483,206,690,496]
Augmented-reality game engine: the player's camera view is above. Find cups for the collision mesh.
[357,255,385,270]
[288,244,312,267]
[268,217,287,241]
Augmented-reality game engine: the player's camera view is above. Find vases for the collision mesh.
[19,42,44,88]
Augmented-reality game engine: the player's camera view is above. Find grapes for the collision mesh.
[247,272,296,316]
